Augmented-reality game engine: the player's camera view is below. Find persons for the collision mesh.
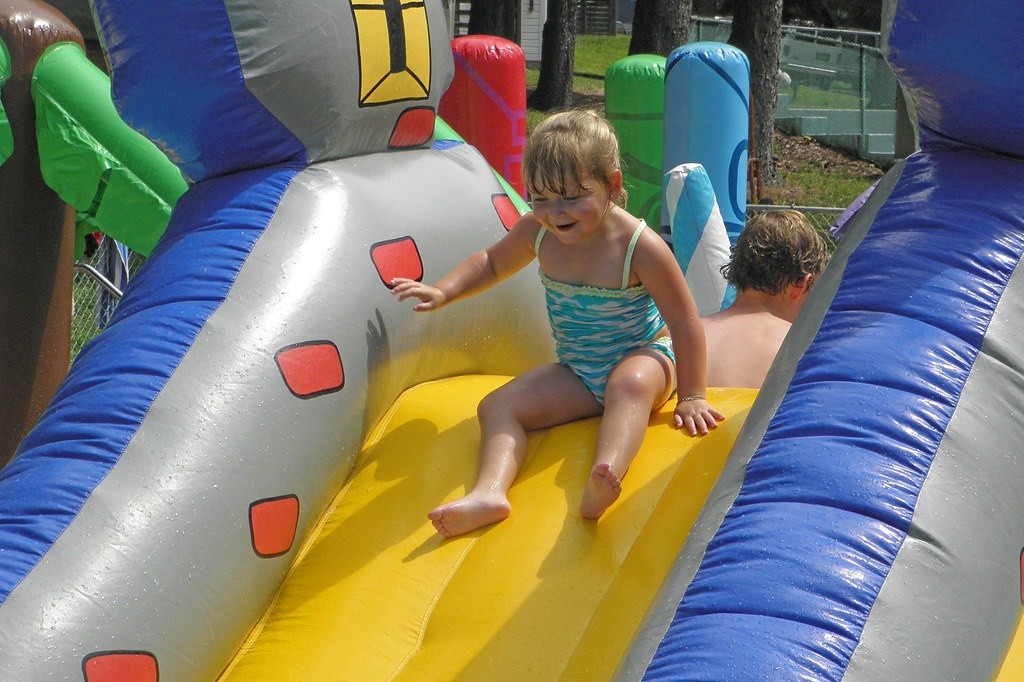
[386,108,726,539]
[653,207,830,389]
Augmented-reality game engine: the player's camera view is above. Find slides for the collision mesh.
[0,197,1024,682]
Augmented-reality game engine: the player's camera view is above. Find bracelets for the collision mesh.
[676,394,706,405]
